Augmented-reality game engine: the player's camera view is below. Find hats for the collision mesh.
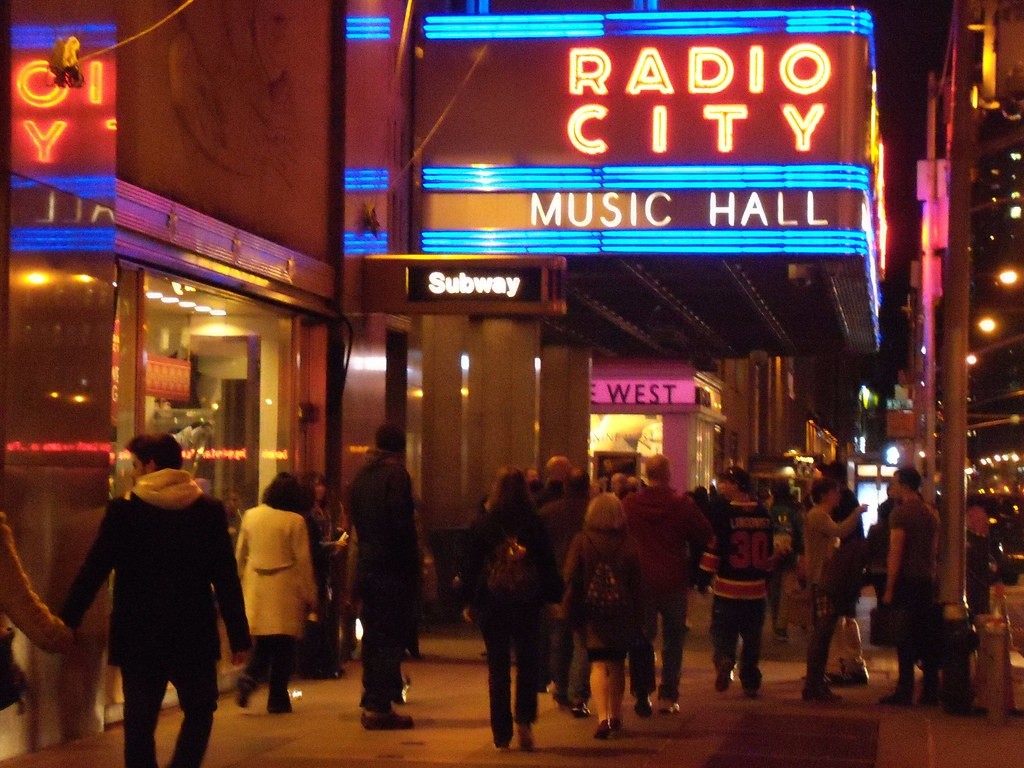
[715,467,753,491]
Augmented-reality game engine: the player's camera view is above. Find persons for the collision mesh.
[0,511,77,710]
[452,450,1018,753]
[236,470,339,713]
[344,423,423,729]
[59,434,252,768]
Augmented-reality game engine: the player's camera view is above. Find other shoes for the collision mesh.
[462,628,994,748]
[235,673,251,708]
[266,703,294,713]
[360,708,415,730]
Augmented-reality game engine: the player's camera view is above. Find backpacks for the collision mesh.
[577,533,634,622]
[477,518,538,603]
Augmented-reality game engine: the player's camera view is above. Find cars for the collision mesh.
[971,491,1024,584]
